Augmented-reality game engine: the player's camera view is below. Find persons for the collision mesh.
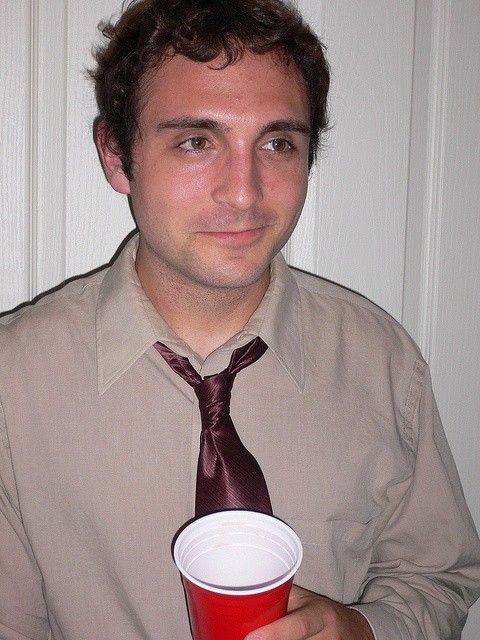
[0,0,480,640]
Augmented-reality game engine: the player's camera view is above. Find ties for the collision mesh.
[154,339,275,524]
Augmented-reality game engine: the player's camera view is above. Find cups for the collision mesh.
[173,510,303,640]
